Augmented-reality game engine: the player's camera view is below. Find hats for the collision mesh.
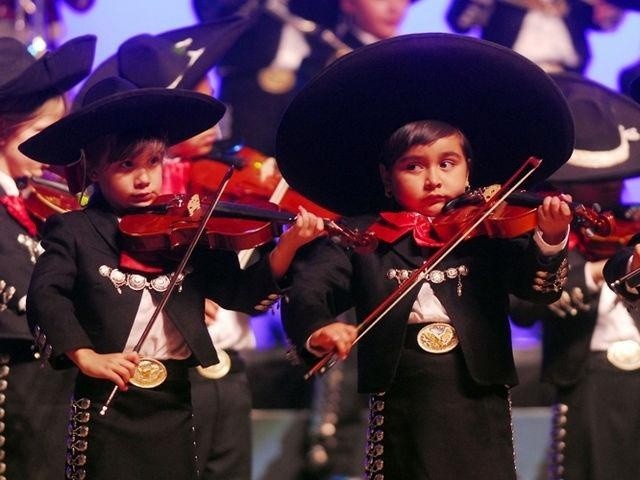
[272,33,575,218]
[71,15,249,113]
[0,34,97,116]
[536,72,639,184]
[18,76,226,166]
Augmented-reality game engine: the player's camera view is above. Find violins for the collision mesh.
[431,185,616,242]
[576,212,640,262]
[18,170,83,223]
[184,138,344,224]
[119,194,374,254]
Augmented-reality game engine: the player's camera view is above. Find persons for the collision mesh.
[245,0,419,120]
[276,33,578,477]
[17,77,324,479]
[0,33,102,479]
[445,2,630,91]
[508,239,640,478]
[78,20,258,477]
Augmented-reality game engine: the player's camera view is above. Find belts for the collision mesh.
[588,340,637,373]
[190,352,243,383]
[131,357,188,392]
[403,324,459,357]
